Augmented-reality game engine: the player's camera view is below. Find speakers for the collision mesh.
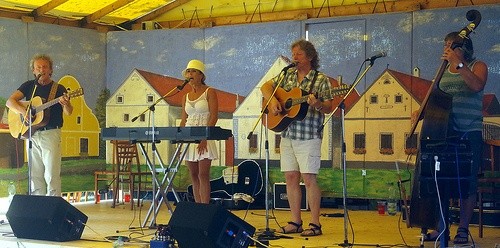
[5,193,88,242]
[168,199,257,248]
[273,181,309,211]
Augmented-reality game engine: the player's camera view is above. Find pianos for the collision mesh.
[100,124,234,229]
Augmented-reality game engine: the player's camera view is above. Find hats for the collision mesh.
[182,60,206,81]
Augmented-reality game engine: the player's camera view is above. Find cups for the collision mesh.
[377,201,386,214]
[123,192,130,202]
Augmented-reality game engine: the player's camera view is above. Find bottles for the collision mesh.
[7,181,16,205]
[97,189,100,203]
[388,182,397,216]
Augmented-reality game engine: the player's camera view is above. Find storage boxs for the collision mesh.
[273,184,310,211]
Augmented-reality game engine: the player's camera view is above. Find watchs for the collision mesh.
[456,62,464,70]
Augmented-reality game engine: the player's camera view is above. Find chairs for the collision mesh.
[476,139,500,238]
[93,141,151,208]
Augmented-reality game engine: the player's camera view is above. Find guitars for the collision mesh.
[261,83,352,133]
[8,87,84,141]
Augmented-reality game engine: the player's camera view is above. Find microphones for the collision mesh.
[180,76,190,92]
[284,62,296,71]
[33,72,43,85]
[369,51,387,64]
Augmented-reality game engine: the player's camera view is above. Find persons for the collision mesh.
[180,60,218,204]
[6,54,73,196]
[261,39,334,236]
[410,32,488,244]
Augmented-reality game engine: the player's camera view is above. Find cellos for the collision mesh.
[395,8,482,248]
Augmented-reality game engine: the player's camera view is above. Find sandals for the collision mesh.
[301,223,322,237]
[453,228,469,244]
[420,230,450,242]
[276,220,304,233]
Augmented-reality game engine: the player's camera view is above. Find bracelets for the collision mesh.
[315,107,323,112]
